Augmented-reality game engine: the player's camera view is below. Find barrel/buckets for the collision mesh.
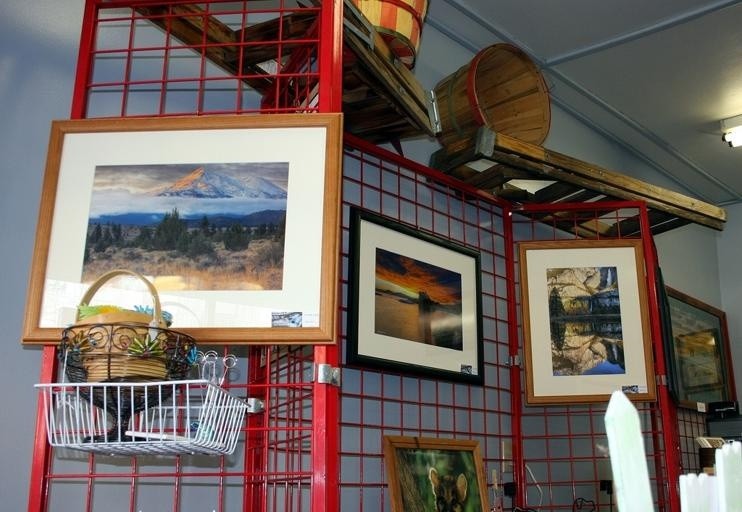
[698,446,719,475]
[433,43,551,147]
[342,0,430,75]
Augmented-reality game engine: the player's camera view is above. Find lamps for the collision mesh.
[719,114,742,149]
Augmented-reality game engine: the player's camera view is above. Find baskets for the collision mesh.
[33,379,251,456]
[57,268,198,443]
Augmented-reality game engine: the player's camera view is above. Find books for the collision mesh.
[694,436,725,448]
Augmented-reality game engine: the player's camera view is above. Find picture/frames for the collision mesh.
[346,202,485,386]
[676,328,725,389]
[22,113,342,346]
[382,435,491,512]
[685,387,726,403]
[516,238,658,408]
[665,284,737,413]
